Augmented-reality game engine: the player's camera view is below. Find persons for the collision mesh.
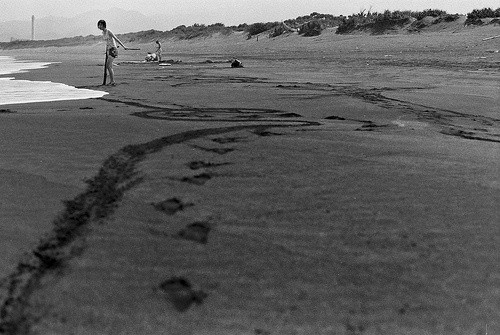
[154,39,163,62]
[96,19,129,87]
